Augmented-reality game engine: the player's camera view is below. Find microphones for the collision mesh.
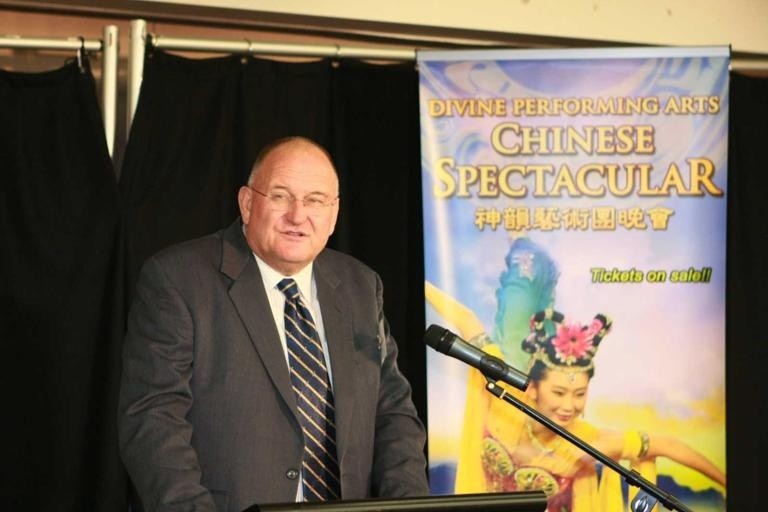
[421,323,532,392]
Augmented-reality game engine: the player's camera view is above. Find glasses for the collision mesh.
[246,182,340,212]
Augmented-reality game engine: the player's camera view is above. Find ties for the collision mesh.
[278,277,342,502]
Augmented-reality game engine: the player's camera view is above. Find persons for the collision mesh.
[117,135,433,512]
[424,229,727,512]
